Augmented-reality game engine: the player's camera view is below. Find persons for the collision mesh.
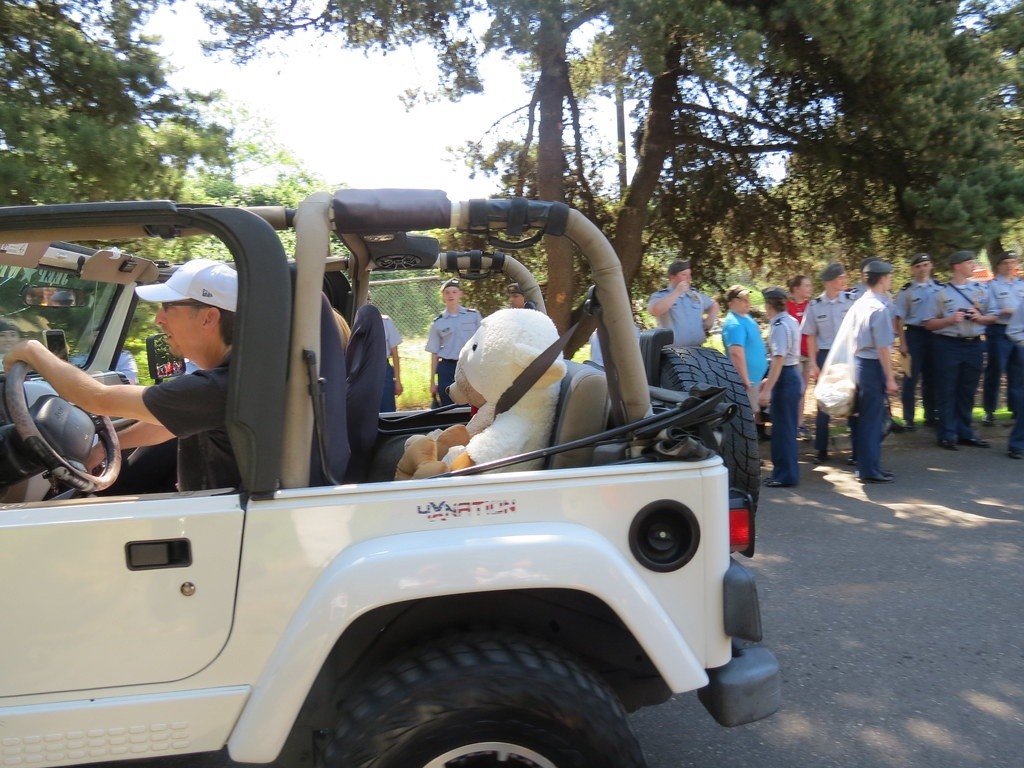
[721,286,768,419]
[365,291,404,414]
[158,361,183,376]
[799,261,858,460]
[852,250,1024,459]
[756,284,804,488]
[68,325,139,385]
[784,273,818,437]
[424,277,484,409]
[848,260,899,483]
[649,260,719,347]
[1,258,244,491]
[507,282,537,309]
[26,287,75,307]
[0,320,22,373]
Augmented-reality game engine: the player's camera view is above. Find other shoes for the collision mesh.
[890,418,942,433]
[1011,411,1018,419]
[982,412,993,426]
[813,454,827,463]
[847,456,856,465]
[1008,446,1024,459]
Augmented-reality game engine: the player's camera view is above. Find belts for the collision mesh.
[782,364,797,369]
[937,437,959,451]
[907,325,931,334]
[441,358,458,364]
[937,334,980,343]
[959,437,991,448]
[387,358,389,363]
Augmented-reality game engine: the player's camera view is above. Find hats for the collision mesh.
[668,260,691,274]
[860,256,891,273]
[727,285,753,303]
[440,278,462,293]
[910,252,931,266]
[49,291,75,302]
[995,250,1016,265]
[948,251,972,263]
[134,259,238,313]
[820,263,844,281]
[507,282,524,293]
[762,287,788,299]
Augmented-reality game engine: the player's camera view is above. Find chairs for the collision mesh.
[312,293,350,488]
[347,304,383,458]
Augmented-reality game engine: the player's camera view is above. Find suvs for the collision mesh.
[0,190,789,768]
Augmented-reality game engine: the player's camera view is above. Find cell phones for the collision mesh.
[42,329,68,362]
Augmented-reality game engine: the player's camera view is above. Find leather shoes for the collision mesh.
[762,477,799,488]
[856,470,896,484]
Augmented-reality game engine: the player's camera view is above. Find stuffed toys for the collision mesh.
[392,308,567,481]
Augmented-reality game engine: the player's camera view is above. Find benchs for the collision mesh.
[374,352,611,478]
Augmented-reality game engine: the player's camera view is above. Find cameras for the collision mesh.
[959,308,972,319]
